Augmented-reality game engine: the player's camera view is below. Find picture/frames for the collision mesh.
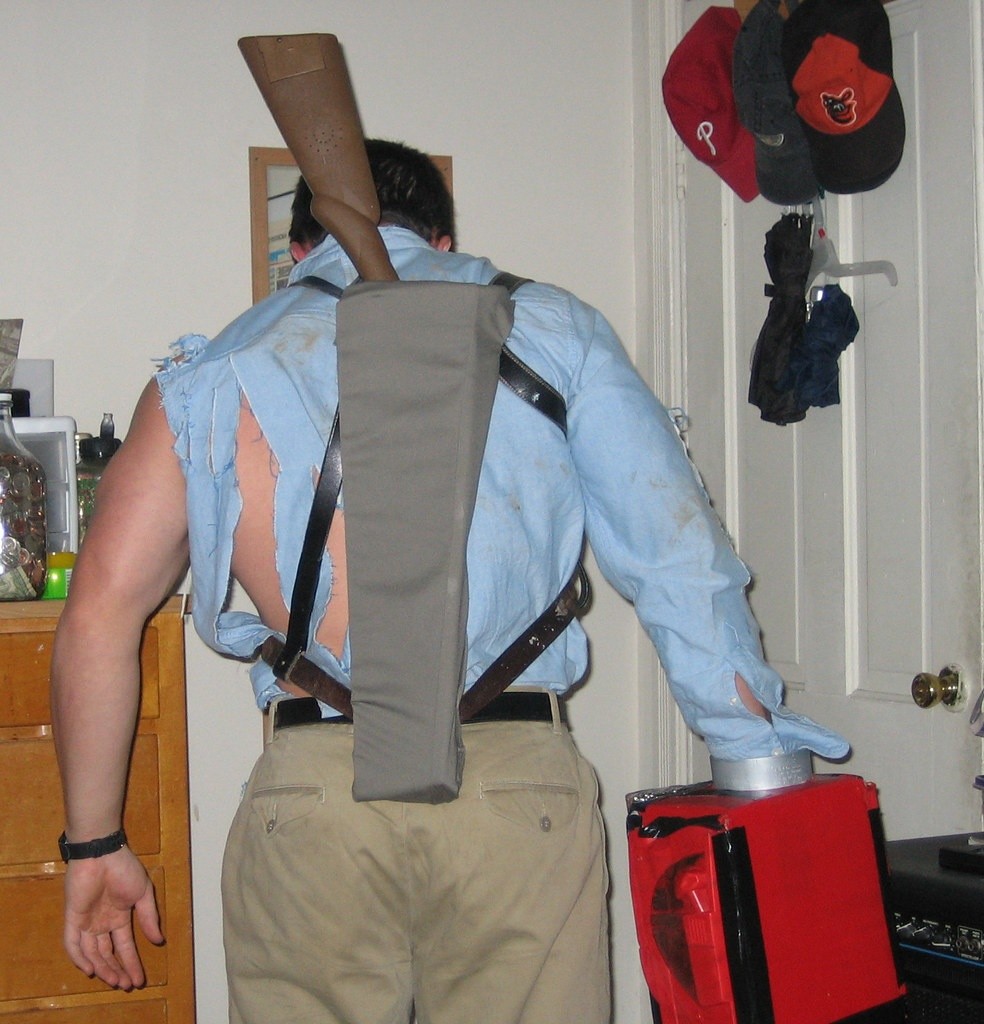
[250,145,453,308]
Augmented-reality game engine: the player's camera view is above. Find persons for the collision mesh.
[41,139,855,1024]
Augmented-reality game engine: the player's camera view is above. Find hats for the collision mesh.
[731,1,827,207]
[782,0,905,197]
[661,7,760,203]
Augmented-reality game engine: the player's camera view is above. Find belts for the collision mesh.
[265,692,570,726]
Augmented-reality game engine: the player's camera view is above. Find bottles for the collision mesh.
[0,393,49,602]
[76,437,123,551]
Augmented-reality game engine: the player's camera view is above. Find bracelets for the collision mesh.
[58,824,128,864]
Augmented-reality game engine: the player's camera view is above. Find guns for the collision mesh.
[233,31,399,283]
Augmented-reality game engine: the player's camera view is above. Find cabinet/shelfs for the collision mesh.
[0,596,195,1024]
[13,416,79,551]
